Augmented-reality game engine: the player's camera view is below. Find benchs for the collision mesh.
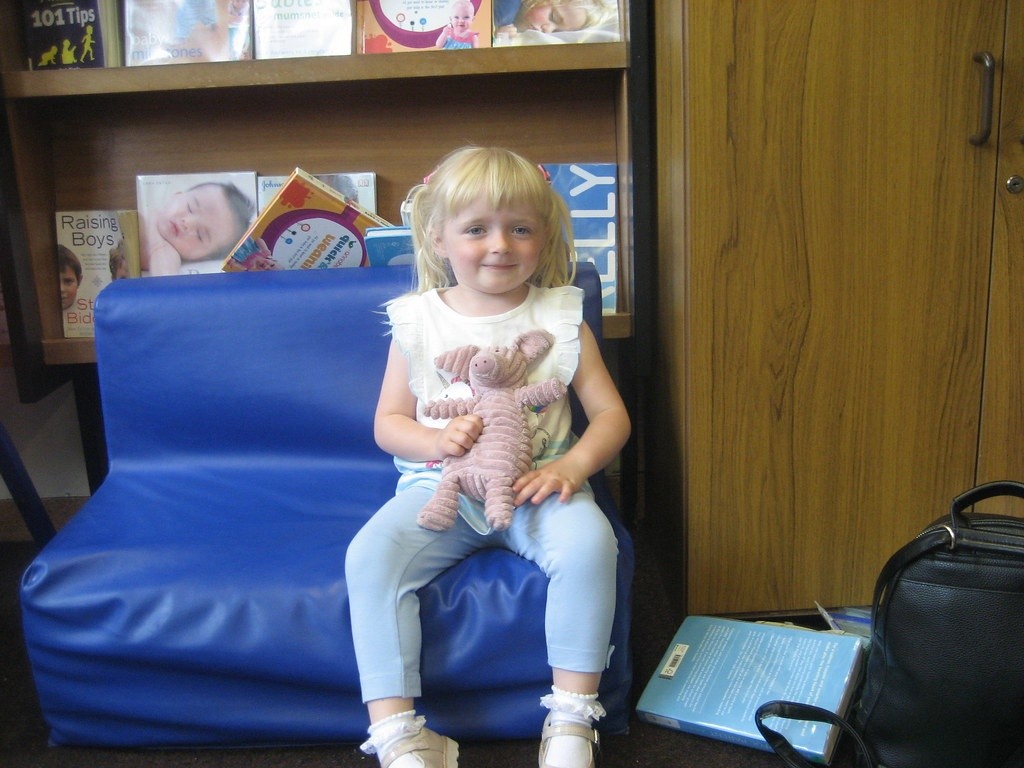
[19,265,639,749]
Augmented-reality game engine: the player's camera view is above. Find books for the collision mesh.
[137,171,258,276]
[54,209,137,337]
[21,0,118,67]
[362,161,621,317]
[635,616,866,763]
[356,0,492,54]
[218,167,395,270]
[252,0,353,59]
[258,175,378,271]
[123,0,249,64]
[492,0,624,49]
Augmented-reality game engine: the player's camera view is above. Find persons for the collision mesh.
[108,241,129,281]
[343,146,633,768]
[137,181,256,276]
[57,245,82,310]
[232,236,279,269]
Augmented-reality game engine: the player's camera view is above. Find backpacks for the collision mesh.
[753,479,1024,768]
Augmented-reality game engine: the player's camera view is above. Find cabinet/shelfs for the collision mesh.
[0,0,635,368]
[646,1,1024,618]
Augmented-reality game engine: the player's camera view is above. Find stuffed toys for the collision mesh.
[417,330,567,533]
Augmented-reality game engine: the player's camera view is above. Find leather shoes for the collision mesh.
[538,711,600,768]
[379,726,460,768]
